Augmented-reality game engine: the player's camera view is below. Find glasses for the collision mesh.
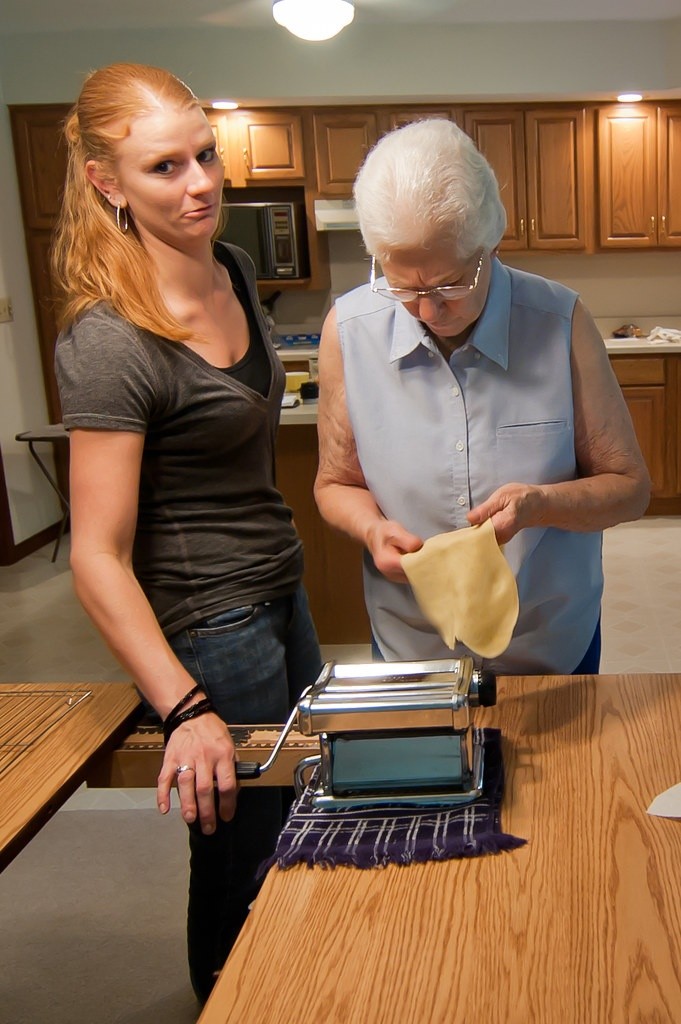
[368,255,486,301]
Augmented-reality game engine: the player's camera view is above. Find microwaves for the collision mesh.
[218,200,300,279]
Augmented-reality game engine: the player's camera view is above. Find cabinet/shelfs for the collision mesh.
[0,91,681,522]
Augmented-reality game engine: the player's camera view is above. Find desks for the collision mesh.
[0,680,144,875]
[198,673,681,1024]
[15,424,72,562]
[271,424,374,645]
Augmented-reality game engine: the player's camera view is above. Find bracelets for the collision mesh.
[163,683,218,745]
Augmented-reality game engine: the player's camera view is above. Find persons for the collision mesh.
[313,118,653,676]
[52,62,324,1008]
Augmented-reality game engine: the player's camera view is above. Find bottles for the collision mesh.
[259,289,283,316]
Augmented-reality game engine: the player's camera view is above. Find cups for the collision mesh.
[285,372,310,392]
[308,358,319,385]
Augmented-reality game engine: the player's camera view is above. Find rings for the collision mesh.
[177,765,195,772]
